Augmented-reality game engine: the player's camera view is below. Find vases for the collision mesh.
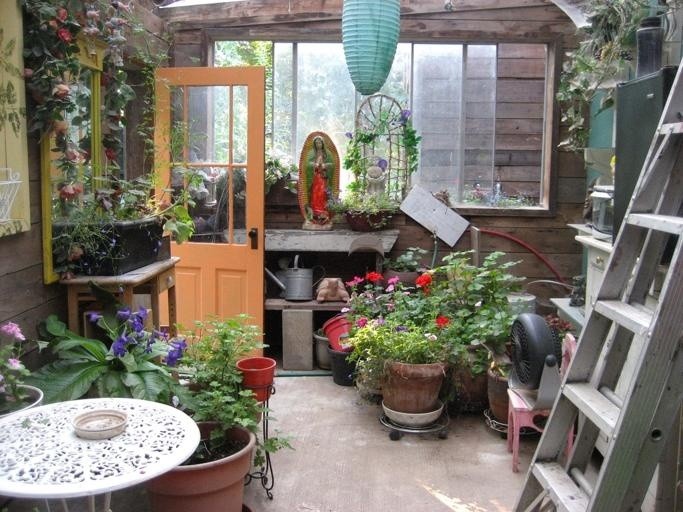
[455,366,488,404]
[0,381,43,420]
[50,219,173,276]
[144,420,256,508]
[488,362,524,423]
[383,359,447,426]
[326,347,357,386]
[311,331,331,369]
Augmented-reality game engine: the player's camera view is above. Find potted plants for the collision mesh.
[161,309,265,438]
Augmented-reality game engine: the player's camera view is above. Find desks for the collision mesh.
[0,398,202,507]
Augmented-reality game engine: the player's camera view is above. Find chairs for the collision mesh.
[506,334,575,473]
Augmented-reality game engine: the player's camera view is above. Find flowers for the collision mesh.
[1,322,47,401]
[344,245,531,363]
[40,280,189,411]
[25,1,131,210]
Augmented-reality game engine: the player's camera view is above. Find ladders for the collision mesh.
[515,56,683,511]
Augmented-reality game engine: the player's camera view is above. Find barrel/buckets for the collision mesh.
[326,346,363,386]
[314,330,336,373]
[323,312,356,351]
